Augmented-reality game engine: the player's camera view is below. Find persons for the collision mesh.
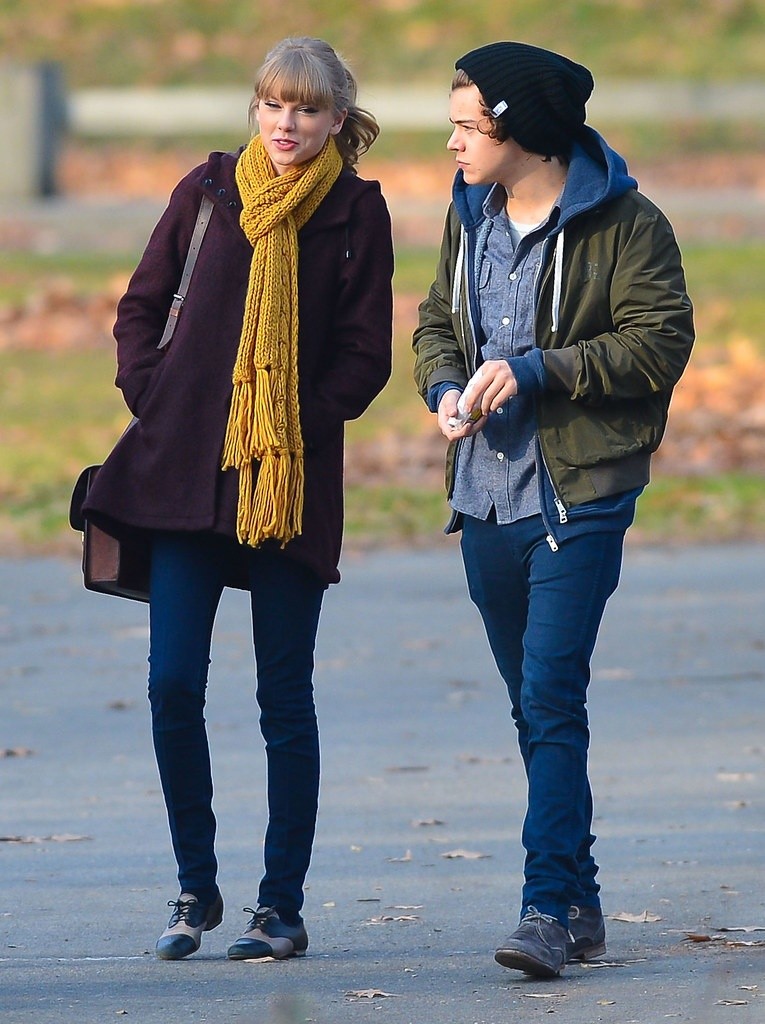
[80,34,395,961]
[412,40,697,983]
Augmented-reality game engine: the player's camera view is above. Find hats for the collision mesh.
[455,42,597,155]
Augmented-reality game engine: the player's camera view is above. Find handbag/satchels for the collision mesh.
[69,467,151,603]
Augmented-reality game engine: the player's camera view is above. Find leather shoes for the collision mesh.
[155,886,225,959]
[228,906,308,960]
[564,905,608,964]
[495,906,568,977]
